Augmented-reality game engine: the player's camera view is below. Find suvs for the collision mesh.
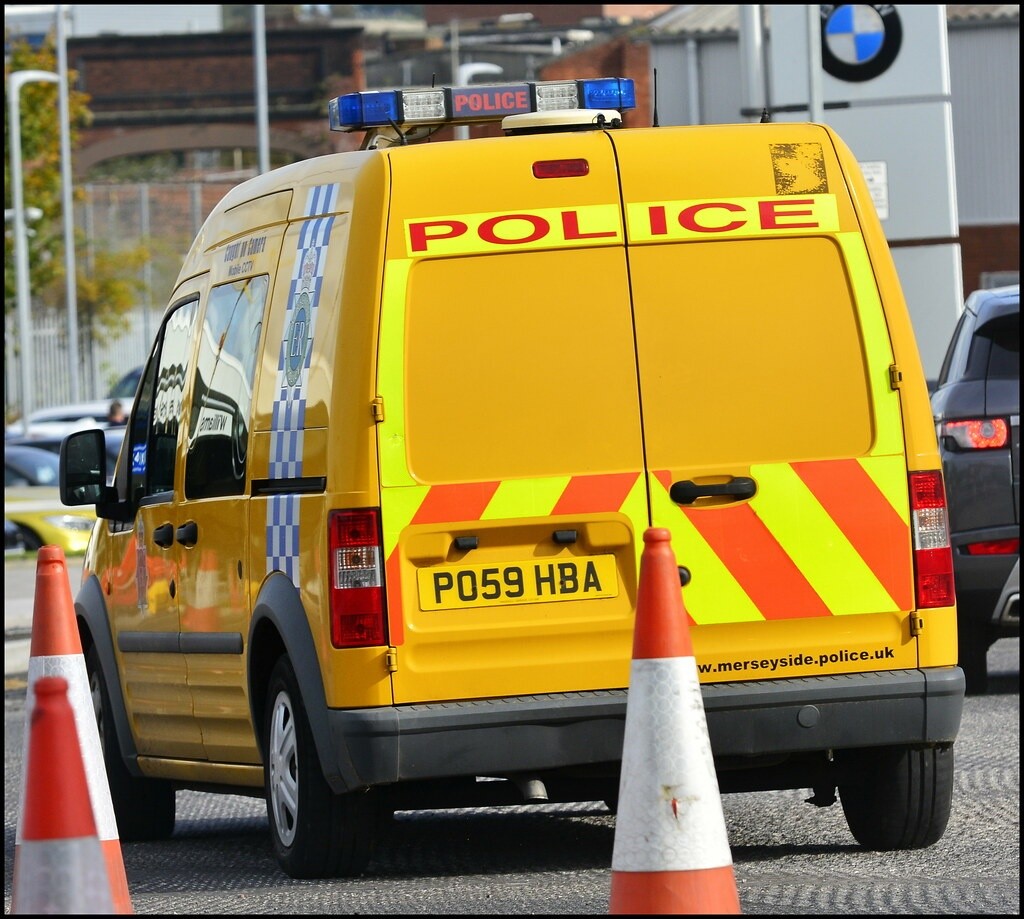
[921,281,1022,678]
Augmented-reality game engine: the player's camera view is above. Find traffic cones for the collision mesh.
[12,675,119,916]
[607,526,741,916]
[9,545,138,919]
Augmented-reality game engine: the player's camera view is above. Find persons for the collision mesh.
[109,404,124,426]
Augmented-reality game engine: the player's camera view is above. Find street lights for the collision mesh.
[457,61,501,142]
[7,66,58,442]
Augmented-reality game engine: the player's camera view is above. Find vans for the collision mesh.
[61,70,971,848]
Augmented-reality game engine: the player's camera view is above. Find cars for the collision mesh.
[5,364,147,555]
